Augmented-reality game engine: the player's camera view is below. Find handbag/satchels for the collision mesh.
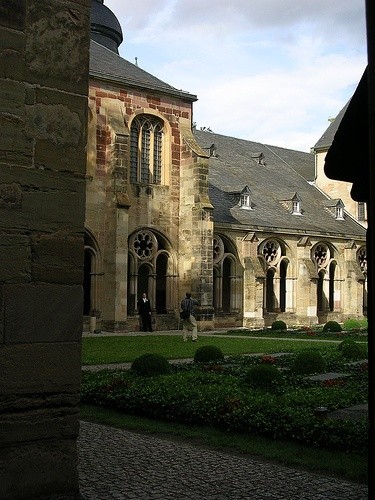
[181,310,190,319]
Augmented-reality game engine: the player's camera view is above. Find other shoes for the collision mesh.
[192,339,197,343]
[184,338,187,342]
[150,329,153,332]
[143,330,146,332]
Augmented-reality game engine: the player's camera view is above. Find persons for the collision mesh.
[181,291,199,343]
[137,292,153,332]
